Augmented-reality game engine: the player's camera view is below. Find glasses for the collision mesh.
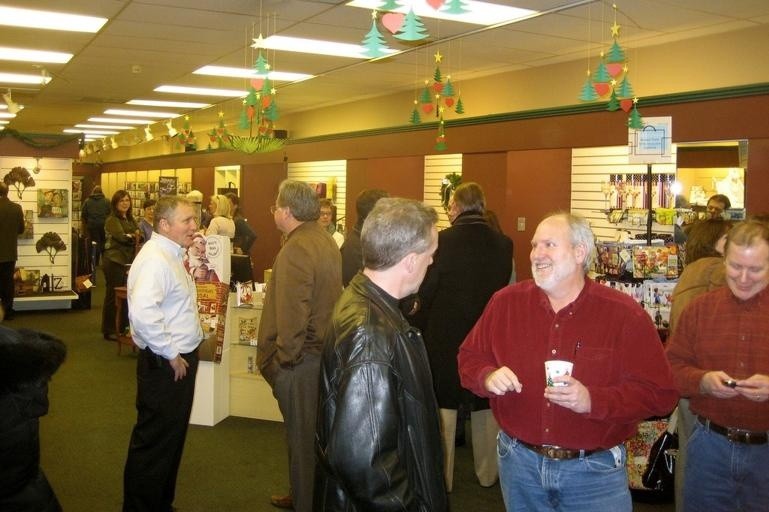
[320,209,332,217]
[269,206,283,213]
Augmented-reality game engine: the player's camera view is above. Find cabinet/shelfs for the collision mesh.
[231,288,295,424]
[71,173,85,232]
[590,164,694,506]
[111,167,242,253]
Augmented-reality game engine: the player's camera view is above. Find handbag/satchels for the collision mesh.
[640,407,680,490]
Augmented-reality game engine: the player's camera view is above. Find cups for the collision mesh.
[544,360,573,389]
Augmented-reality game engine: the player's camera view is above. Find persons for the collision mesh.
[663,219,769,512]
[314,197,345,251]
[269,179,342,512]
[669,215,734,338]
[706,194,731,217]
[456,211,681,512]
[340,189,391,288]
[313,198,448,512]
[408,180,513,493]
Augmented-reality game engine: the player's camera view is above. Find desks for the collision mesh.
[110,283,137,357]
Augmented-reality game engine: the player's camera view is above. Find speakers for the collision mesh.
[186,144,196,151]
[272,130,288,139]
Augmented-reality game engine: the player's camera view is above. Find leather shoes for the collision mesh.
[104,333,117,340]
[270,494,293,508]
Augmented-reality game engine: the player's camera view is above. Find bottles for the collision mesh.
[42,274,49,293]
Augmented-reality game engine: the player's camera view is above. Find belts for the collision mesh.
[505,433,604,461]
[694,415,767,446]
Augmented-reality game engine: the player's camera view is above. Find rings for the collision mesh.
[756,396,760,401]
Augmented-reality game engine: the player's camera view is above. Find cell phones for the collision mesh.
[723,380,736,387]
